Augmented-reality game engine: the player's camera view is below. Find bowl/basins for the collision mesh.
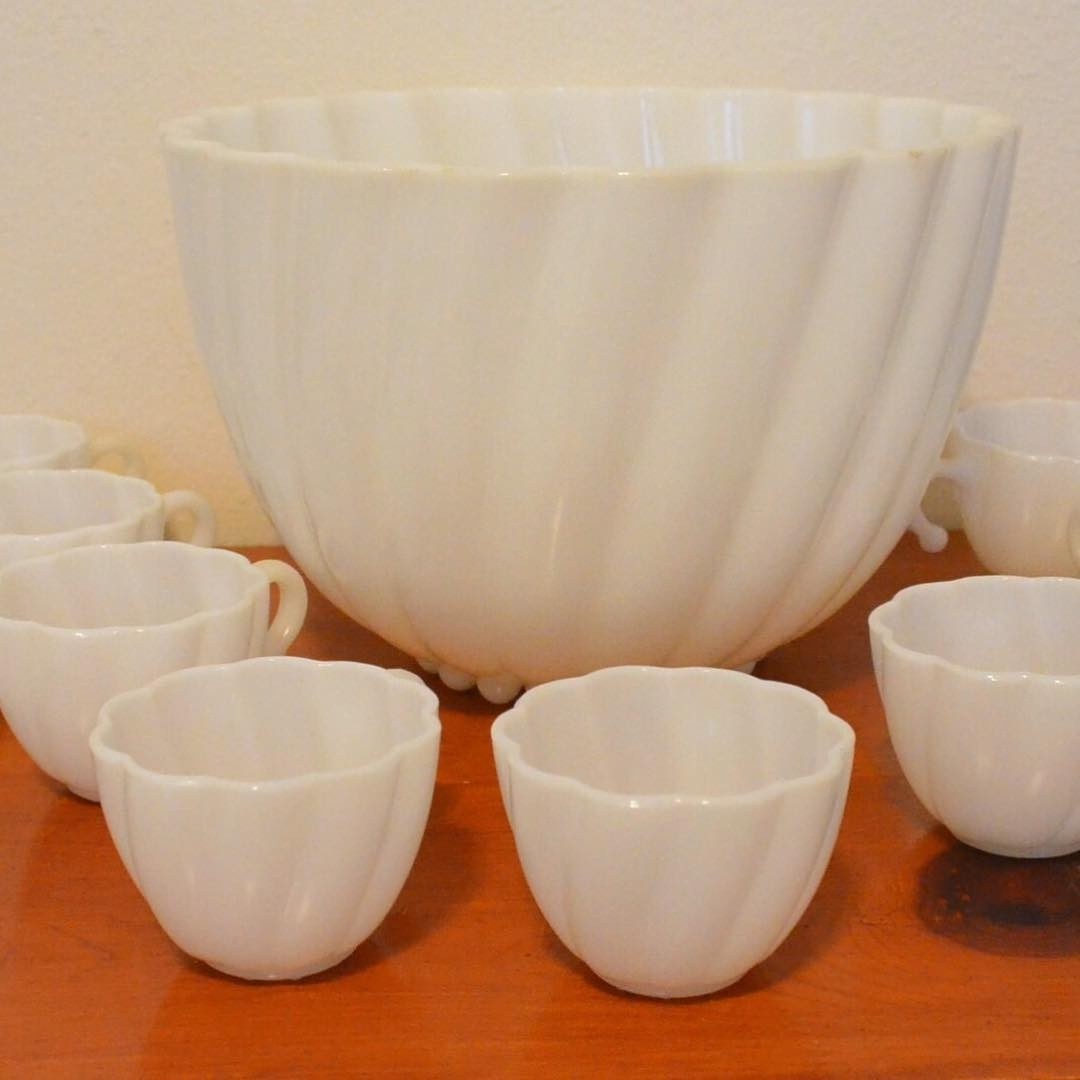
[146,80,1014,688]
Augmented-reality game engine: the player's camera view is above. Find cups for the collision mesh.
[0,414,88,477]
[89,656,444,982]
[0,540,311,802]
[487,662,859,999]
[866,572,1080,862]
[901,398,1080,580]
[0,469,215,577]
